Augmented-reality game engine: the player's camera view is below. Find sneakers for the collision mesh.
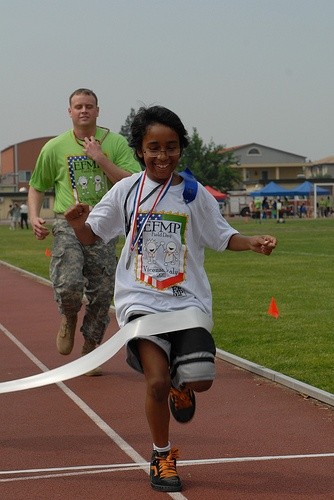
[81,344,103,376]
[150,448,183,492]
[56,314,77,355]
[169,381,196,424]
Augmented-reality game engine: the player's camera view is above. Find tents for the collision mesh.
[203,186,228,199]
[248,181,329,196]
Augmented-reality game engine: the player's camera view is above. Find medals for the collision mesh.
[126,255,133,271]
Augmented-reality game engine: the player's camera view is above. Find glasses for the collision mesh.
[141,147,180,157]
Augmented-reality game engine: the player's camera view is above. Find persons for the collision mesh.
[64,106,278,493]
[28,88,142,376]
[7,196,331,231]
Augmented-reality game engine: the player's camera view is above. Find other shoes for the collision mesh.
[276,220,285,223]
